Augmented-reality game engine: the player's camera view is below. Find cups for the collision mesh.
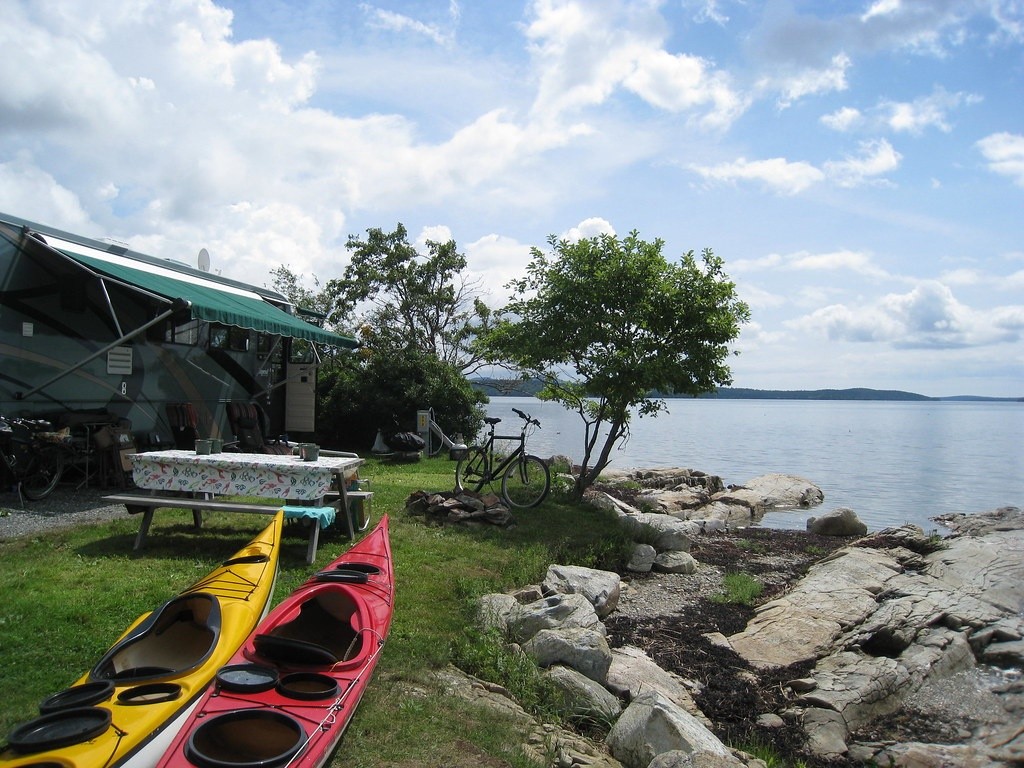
[207,438,224,453]
[195,439,212,455]
[302,445,320,461]
[299,443,315,458]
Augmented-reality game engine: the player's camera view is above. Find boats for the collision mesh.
[0,508,285,768]
[174,512,390,768]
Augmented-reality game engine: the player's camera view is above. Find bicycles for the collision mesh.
[0,414,66,510]
[456,408,551,511]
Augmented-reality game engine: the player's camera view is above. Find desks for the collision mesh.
[123,450,368,563]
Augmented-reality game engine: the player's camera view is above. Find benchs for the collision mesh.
[101,493,339,520]
[325,489,374,500]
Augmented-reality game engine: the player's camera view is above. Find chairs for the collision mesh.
[165,402,201,450]
[226,402,292,455]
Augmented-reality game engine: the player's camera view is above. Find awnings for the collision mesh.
[30,230,365,349]
